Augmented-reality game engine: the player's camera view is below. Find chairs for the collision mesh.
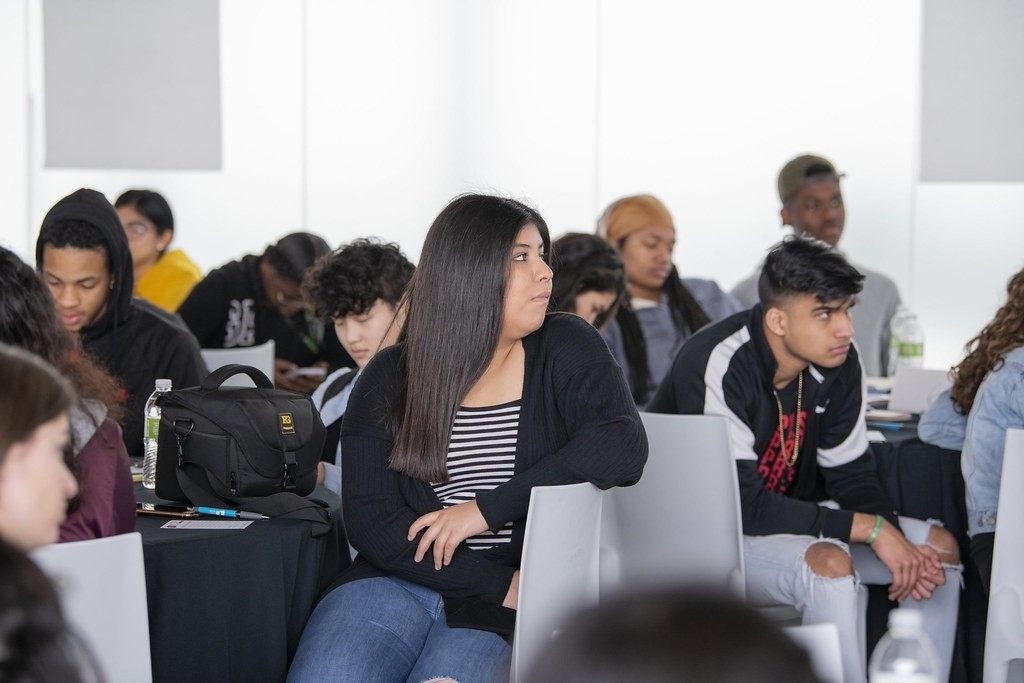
[27,342,1024,683]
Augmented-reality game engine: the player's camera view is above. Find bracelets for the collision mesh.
[865,514,883,546]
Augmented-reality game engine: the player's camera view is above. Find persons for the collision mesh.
[286,182,649,683]
[918,264,1024,612]
[729,156,902,377]
[36,188,211,458]
[176,232,360,463]
[316,234,416,561]
[548,232,626,332]
[0,244,137,683]
[642,230,964,683]
[594,195,741,412]
[113,189,206,314]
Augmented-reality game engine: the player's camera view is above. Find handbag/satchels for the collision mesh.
[155,364,333,536]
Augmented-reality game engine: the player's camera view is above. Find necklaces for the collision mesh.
[773,373,803,467]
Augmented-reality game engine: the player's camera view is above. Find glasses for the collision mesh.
[122,221,160,236]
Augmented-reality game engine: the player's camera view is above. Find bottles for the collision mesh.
[869,608,940,683]
[142,378,174,489]
[887,304,924,376]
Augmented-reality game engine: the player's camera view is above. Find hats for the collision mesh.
[777,155,845,203]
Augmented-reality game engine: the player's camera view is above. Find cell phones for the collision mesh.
[135,501,203,518]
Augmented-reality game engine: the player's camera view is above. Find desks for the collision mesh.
[129,454,341,683]
[860,421,976,683]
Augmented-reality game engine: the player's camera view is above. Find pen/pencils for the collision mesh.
[865,421,918,430]
[187,506,270,519]
